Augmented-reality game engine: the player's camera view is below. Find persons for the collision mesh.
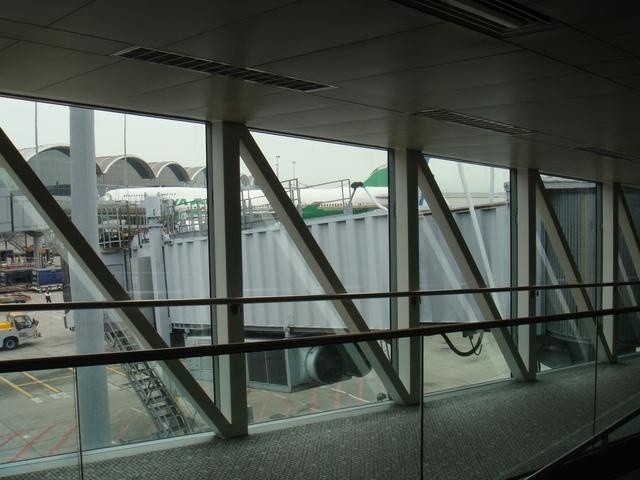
[45,290,51,303]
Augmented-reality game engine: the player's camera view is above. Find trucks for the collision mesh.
[0,310,41,353]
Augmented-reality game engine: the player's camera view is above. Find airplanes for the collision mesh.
[97,161,390,228]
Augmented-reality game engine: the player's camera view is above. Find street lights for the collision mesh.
[275,154,281,177]
[291,160,298,180]
